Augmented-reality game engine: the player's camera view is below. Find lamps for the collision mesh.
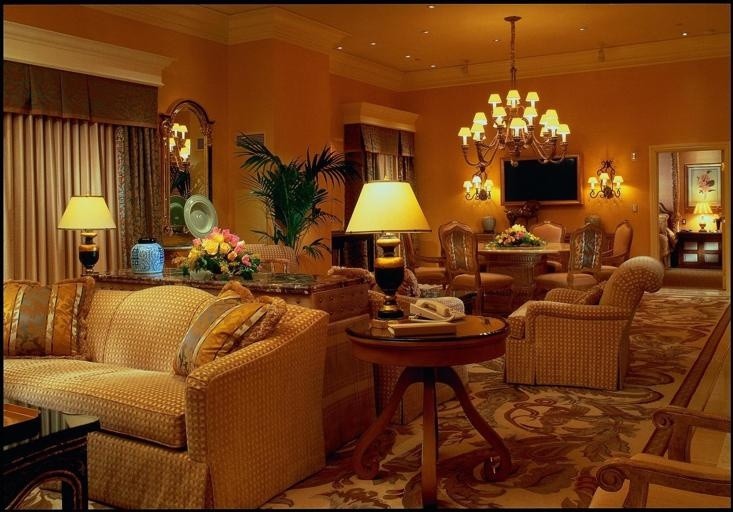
[168,121,190,199]
[55,192,118,277]
[586,159,627,200]
[462,163,494,201]
[693,202,714,232]
[342,173,434,332]
[455,15,573,168]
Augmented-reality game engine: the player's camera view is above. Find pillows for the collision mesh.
[171,278,290,378]
[3,274,97,363]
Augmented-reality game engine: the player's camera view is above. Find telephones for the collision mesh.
[409,299,466,321]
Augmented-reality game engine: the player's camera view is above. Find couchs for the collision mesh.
[2,283,335,510]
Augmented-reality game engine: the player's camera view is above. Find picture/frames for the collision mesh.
[682,161,724,215]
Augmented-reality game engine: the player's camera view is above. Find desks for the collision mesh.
[3,394,103,509]
[341,312,514,508]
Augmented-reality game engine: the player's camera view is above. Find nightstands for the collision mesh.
[676,231,723,271]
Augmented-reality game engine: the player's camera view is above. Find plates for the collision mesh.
[183,194,217,239]
[407,315,455,323]
[168,195,186,226]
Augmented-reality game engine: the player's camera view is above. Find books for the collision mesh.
[388,319,455,337]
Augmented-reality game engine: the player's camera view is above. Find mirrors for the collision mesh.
[158,96,217,240]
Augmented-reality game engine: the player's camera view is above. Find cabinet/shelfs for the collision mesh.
[94,267,380,466]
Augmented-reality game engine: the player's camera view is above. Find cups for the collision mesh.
[480,216,496,234]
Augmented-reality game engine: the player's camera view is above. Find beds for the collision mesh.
[658,201,679,271]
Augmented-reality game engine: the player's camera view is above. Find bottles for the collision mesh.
[584,212,600,227]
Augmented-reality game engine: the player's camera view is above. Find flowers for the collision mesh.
[169,226,265,282]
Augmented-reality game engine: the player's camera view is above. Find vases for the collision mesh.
[479,215,497,234]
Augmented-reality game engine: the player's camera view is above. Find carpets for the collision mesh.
[0,285,730,509]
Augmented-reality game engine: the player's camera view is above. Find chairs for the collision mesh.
[437,219,634,316]
[245,242,299,274]
[501,253,665,394]
[328,265,470,426]
[595,406,731,508]
[401,233,449,291]
